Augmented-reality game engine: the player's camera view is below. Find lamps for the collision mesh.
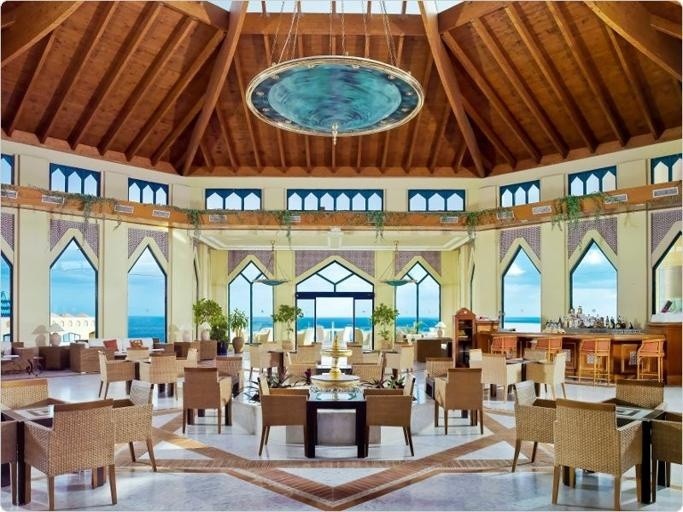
[246,0,426,146]
